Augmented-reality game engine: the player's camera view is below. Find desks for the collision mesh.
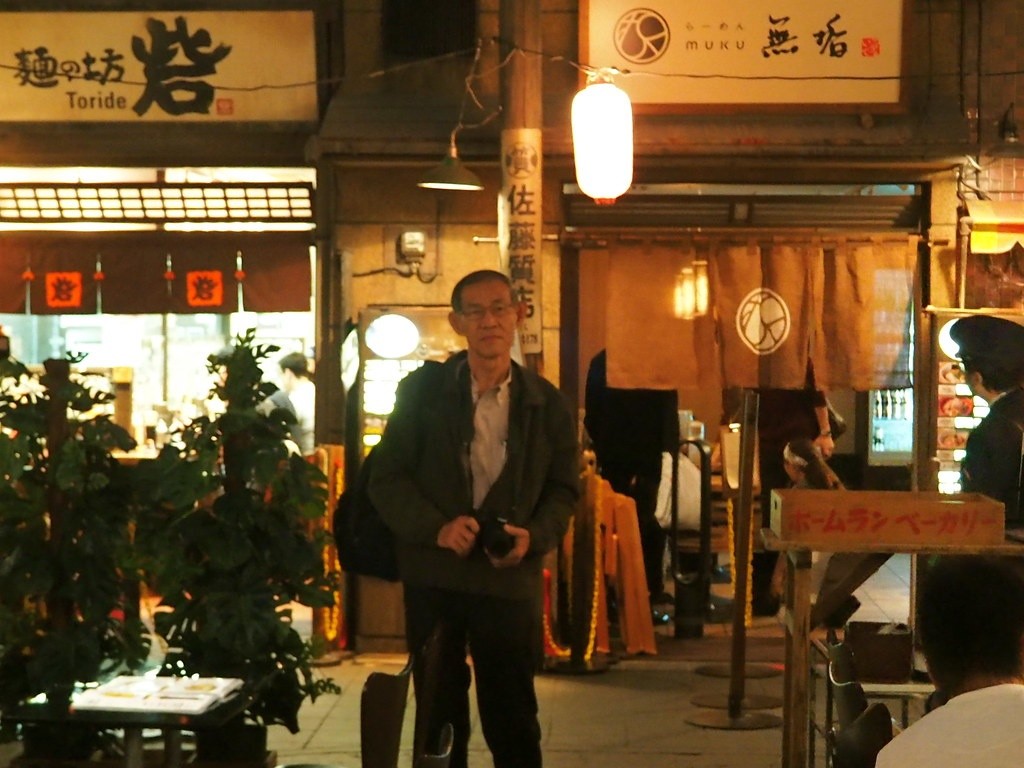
[759,527,1024,768]
[0,681,258,768]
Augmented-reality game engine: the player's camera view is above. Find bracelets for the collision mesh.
[821,431,832,437]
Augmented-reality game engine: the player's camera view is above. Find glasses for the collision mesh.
[951,364,975,379]
[459,303,515,320]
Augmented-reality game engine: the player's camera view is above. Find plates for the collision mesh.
[937,364,976,451]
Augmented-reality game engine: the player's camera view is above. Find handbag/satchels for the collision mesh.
[332,361,446,582]
[827,403,846,439]
[655,451,702,531]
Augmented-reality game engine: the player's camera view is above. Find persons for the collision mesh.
[364,270,584,768]
[585,345,687,624]
[214,345,315,456]
[756,356,849,615]
[874,553,1024,768]
[950,315,1024,529]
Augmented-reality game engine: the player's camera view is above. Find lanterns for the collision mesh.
[571,83,634,205]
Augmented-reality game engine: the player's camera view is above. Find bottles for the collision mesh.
[873,387,912,451]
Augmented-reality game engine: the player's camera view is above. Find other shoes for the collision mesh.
[713,565,731,583]
[651,607,669,626]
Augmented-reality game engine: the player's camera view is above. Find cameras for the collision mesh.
[469,511,513,556]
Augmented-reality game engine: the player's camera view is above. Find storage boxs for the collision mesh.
[768,486,1006,548]
[843,621,913,683]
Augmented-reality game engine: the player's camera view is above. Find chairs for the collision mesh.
[828,639,893,767]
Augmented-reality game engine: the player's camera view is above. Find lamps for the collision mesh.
[415,106,504,192]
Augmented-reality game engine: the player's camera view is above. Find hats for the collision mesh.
[950,315,1024,385]
